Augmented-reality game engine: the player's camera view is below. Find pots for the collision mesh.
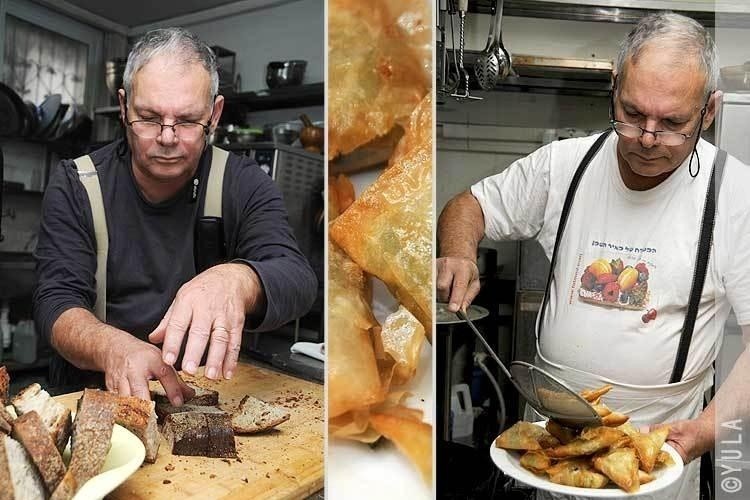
[298,112,324,153]
[265,60,307,90]
[262,121,302,147]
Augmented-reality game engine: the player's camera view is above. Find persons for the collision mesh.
[435,10,750,500]
[34,25,320,410]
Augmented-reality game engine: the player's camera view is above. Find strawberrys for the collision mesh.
[580,257,649,303]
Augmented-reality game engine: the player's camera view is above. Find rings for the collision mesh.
[210,325,229,337]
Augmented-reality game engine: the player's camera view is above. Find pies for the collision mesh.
[331,2,432,484]
[496,384,677,495]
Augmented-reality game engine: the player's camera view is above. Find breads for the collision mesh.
[160,411,236,459]
[115,397,160,463]
[0,366,115,500]
[164,381,220,406]
[230,394,289,434]
[154,402,225,426]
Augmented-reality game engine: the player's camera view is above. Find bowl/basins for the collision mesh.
[104,57,127,106]
[226,131,260,144]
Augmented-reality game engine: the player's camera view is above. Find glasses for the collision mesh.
[609,72,702,148]
[123,91,217,143]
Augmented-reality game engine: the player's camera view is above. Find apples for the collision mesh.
[642,314,650,323]
[646,308,656,320]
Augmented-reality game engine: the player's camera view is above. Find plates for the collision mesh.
[0,82,71,145]
[60,412,146,500]
[489,421,684,498]
